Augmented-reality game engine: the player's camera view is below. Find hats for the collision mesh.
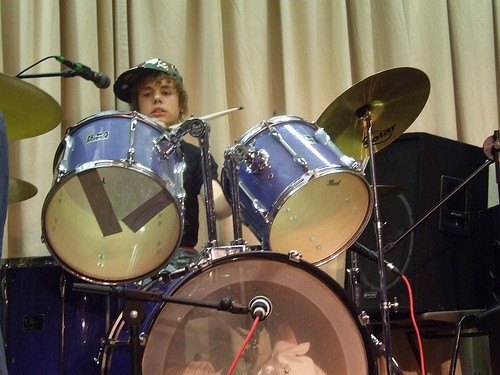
[113,58,180,104]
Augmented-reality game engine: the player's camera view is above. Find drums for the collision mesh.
[36,110,185,285]
[219,114,374,267]
[101,250,376,375]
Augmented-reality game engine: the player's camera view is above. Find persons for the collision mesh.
[114,58,232,286]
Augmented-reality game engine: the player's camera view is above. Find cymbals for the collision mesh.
[0,72,62,141]
[8,175,38,204]
[316,67,431,160]
